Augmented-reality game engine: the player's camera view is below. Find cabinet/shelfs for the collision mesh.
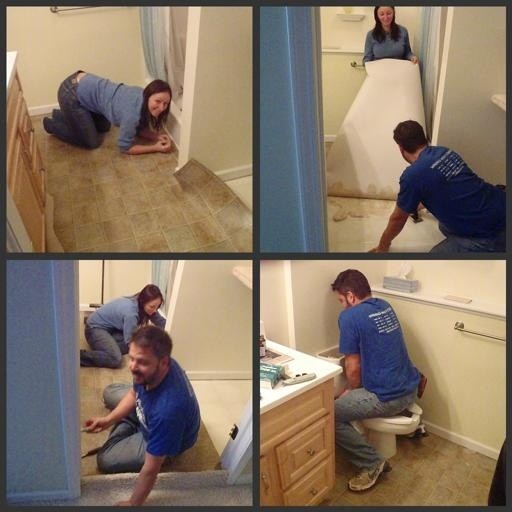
[6,69,49,254]
[260,377,336,505]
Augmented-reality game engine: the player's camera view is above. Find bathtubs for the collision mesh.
[146,73,185,149]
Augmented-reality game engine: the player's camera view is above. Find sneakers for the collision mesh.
[347,460,385,492]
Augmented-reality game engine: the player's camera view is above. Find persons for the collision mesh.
[368,120,506,254]
[79,284,167,367]
[362,6,421,66]
[316,267,427,492]
[81,325,201,506]
[43,69,173,154]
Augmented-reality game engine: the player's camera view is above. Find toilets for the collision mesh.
[318,346,424,461]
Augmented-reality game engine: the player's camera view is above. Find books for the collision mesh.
[260,362,286,390]
